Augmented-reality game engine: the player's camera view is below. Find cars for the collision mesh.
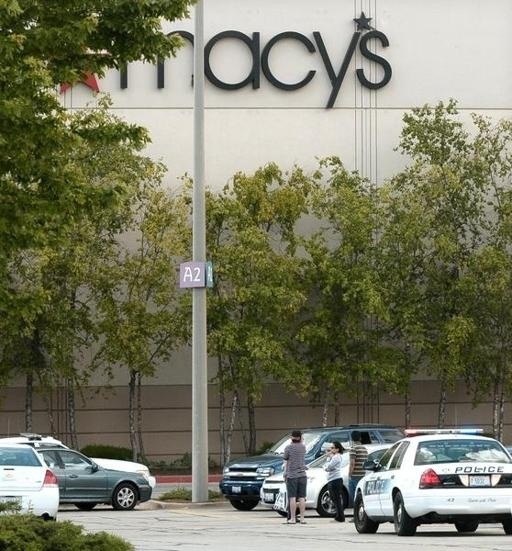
[0,433,157,520]
[260,443,393,518]
[352,428,512,537]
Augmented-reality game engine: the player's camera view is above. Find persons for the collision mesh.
[324,441,345,523]
[348,430,368,523]
[281,431,308,524]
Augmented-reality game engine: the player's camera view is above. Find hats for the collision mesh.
[290,432,301,437]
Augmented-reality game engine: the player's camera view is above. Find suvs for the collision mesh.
[219,424,403,510]
